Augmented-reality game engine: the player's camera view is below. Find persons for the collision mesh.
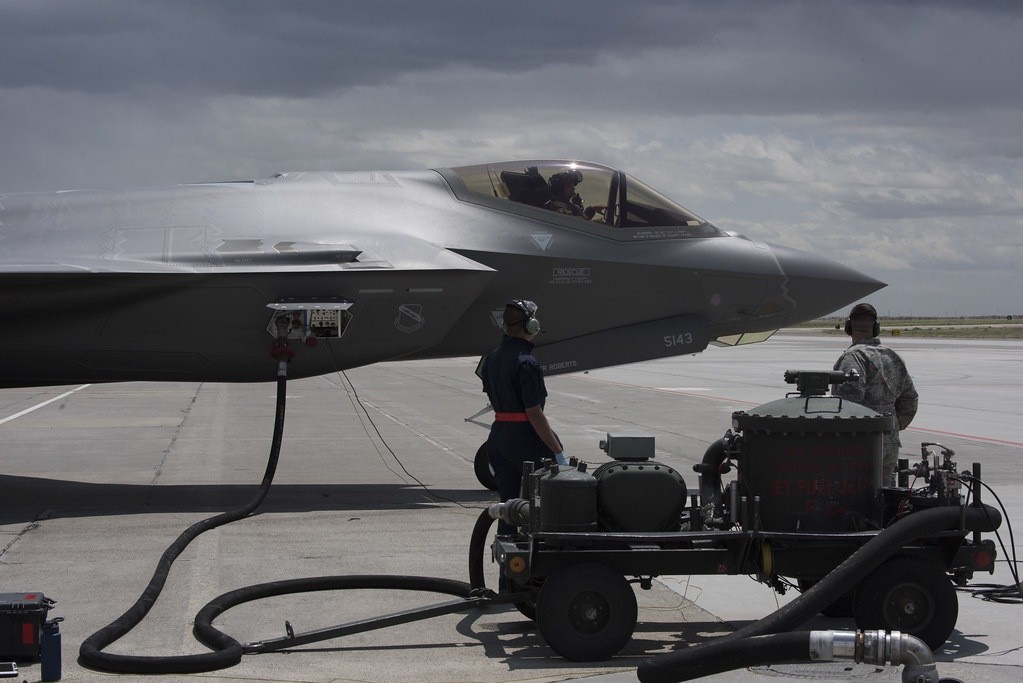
[831,303,919,486]
[482,299,568,536]
[547,173,606,220]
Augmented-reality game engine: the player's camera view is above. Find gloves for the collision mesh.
[555,451,570,468]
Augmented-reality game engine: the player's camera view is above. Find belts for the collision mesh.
[494,411,528,422]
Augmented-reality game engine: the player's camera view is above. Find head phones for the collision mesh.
[500,300,540,337]
[845,303,880,338]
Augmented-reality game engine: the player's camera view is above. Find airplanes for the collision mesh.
[1,155,892,390]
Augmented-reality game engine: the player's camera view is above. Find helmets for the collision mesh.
[549,169,583,193]
